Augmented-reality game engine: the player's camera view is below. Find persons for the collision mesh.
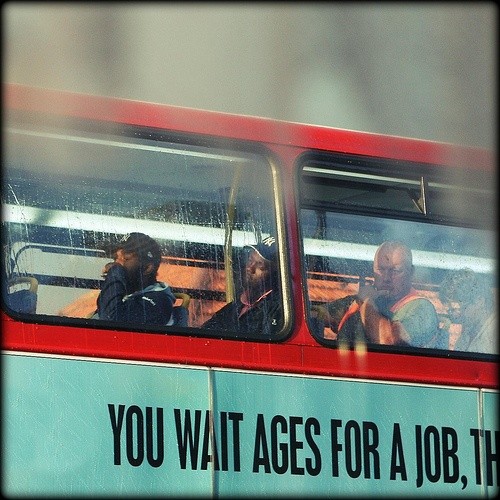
[200,236,285,334]
[308,240,439,347]
[89,233,177,326]
[439,267,500,354]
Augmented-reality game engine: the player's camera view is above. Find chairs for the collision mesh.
[168,293,190,327]
[309,305,325,338]
[436,315,451,351]
[5,277,38,314]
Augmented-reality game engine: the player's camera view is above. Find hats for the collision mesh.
[103,233,160,267]
[246,237,282,264]
